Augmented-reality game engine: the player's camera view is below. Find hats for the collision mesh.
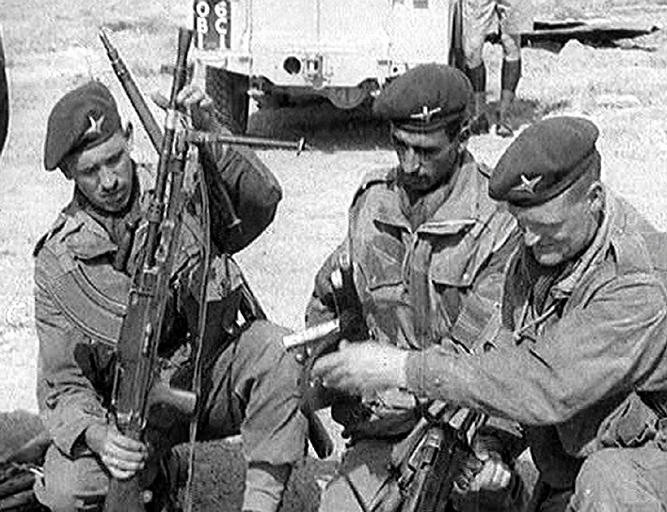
[489,117,599,209]
[44,82,119,171]
[374,64,473,134]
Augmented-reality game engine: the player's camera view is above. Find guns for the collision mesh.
[105,26,308,512]
[364,301,526,512]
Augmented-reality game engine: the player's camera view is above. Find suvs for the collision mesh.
[192,0,522,135]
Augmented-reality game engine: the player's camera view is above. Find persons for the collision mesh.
[29,81,312,512]
[458,0,523,134]
[296,59,526,512]
[314,115,667,511]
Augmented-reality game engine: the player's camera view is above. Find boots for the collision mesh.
[497,59,519,136]
[467,63,489,127]
